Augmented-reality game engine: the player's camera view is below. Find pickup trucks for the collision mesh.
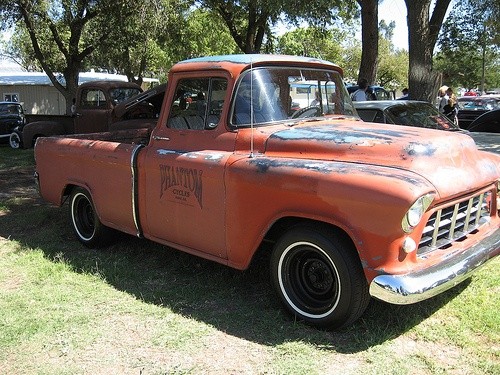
[32,56,500,333]
[22,80,144,150]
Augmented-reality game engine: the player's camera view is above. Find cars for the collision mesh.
[464,89,496,96]
[305,100,477,143]
[346,86,394,100]
[457,97,500,129]
[0,101,26,139]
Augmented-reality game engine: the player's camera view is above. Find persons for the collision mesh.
[396,87,409,100]
[436,86,461,125]
[349,78,369,102]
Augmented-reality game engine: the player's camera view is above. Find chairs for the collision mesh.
[172,113,220,130]
